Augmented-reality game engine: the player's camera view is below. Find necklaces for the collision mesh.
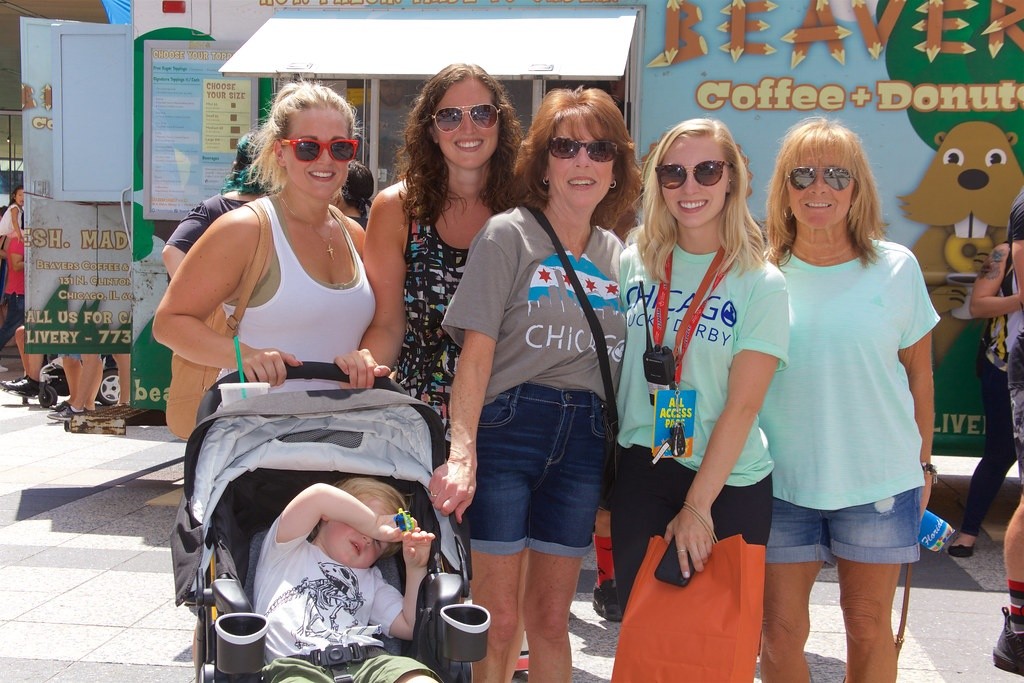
[278,189,335,262]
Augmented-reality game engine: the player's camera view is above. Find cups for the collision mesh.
[217,382,271,407]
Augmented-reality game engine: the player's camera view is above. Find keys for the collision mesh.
[669,422,687,457]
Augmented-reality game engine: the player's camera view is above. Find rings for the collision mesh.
[676,550,689,554]
[430,490,438,497]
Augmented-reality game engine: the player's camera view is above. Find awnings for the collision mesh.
[222,14,642,80]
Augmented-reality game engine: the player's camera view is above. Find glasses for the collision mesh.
[282,139,358,163]
[788,167,854,191]
[431,103,500,134]
[655,160,733,190]
[549,136,617,163]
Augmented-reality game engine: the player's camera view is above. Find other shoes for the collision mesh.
[49,400,71,412]
[948,537,975,557]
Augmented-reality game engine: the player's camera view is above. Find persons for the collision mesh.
[161,133,273,286]
[993,185,1024,674]
[252,476,442,683]
[334,159,374,229]
[0,354,130,422]
[0,326,46,397]
[429,87,646,683]
[946,241,1017,558]
[609,119,790,683]
[593,481,623,618]
[334,63,534,682]
[0,184,24,372]
[151,82,376,390]
[761,120,936,683]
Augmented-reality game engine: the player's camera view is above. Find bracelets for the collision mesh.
[920,461,938,484]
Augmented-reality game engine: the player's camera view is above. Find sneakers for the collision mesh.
[0,376,39,400]
[47,407,74,422]
[593,579,622,620]
[992,607,1024,676]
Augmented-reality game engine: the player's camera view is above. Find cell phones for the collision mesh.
[655,535,695,586]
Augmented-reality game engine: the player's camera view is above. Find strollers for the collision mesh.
[170,360,491,683]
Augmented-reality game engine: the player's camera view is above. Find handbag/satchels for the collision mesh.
[165,301,229,444]
[600,401,619,511]
[611,502,766,683]
